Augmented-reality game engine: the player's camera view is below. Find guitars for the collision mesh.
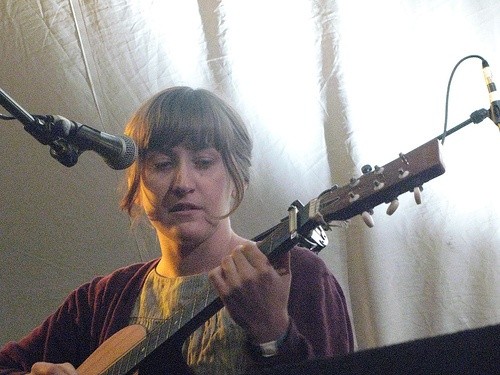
[76,138,446,375]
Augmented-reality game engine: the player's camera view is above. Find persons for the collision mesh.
[0,86,355,375]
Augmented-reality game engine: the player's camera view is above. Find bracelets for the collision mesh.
[242,318,292,359]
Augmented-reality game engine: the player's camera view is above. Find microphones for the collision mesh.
[44,114,138,170]
[482,60,500,130]
